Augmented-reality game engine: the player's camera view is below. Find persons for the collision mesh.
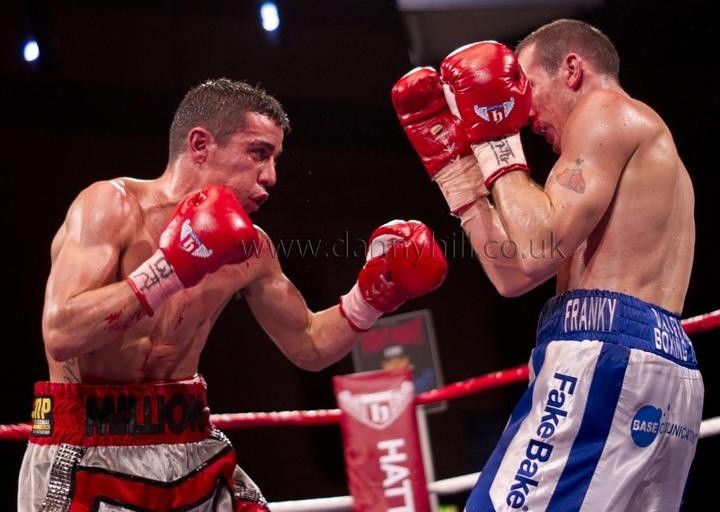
[391,20,706,511]
[18,80,446,511]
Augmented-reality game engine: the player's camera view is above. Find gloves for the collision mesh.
[338,220,449,334]
[123,185,262,317]
[391,66,490,215]
[440,40,534,189]
